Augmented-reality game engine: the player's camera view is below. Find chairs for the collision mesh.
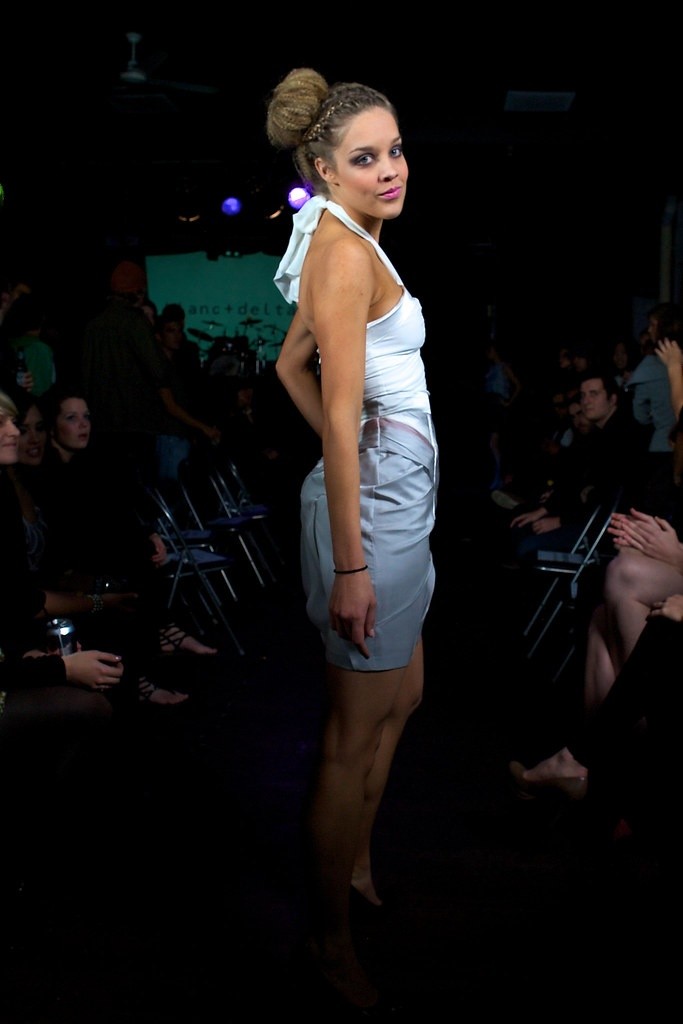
[221,455,285,567]
[150,494,244,656]
[203,467,276,588]
[153,488,238,606]
[522,498,618,662]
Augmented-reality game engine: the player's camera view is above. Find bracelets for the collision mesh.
[334,564,368,574]
[150,533,159,540]
[86,593,103,614]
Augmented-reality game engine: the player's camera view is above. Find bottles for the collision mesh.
[16,345,29,393]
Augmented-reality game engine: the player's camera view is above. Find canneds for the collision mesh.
[46,618,76,657]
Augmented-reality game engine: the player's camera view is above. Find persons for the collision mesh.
[267,67,440,1024]
[0,184,282,726]
[480,302,683,801]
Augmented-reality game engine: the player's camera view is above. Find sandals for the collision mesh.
[125,675,192,715]
[158,623,218,670]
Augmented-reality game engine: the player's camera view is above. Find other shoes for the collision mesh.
[311,866,390,924]
[320,973,404,1024]
[506,759,590,811]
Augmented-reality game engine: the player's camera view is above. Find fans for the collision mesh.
[118,32,217,94]
